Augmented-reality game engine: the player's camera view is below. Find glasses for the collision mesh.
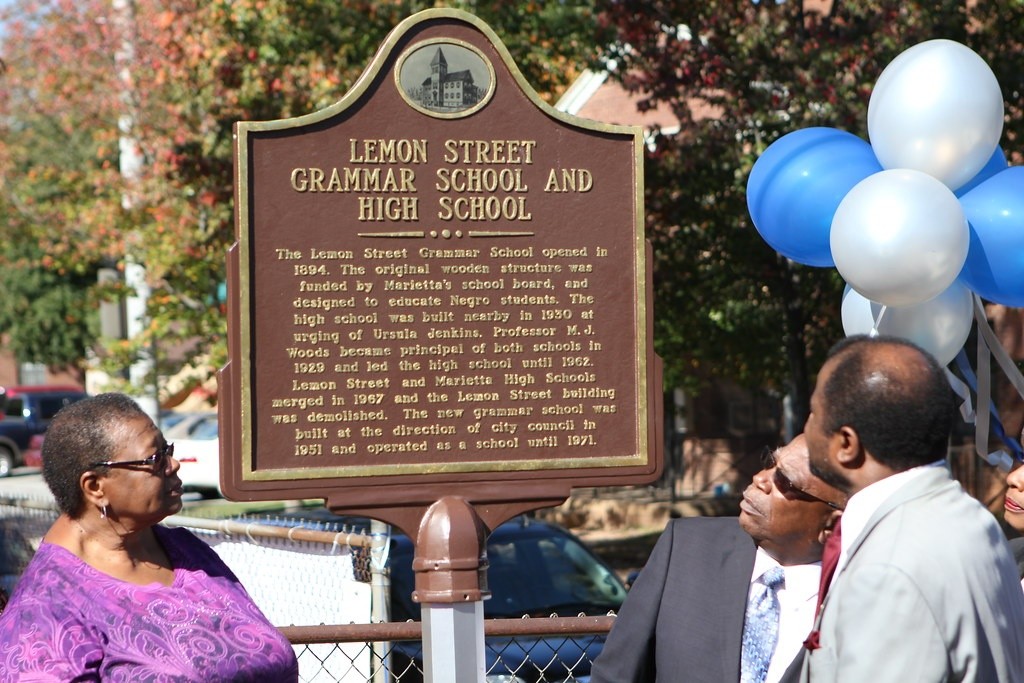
[759,445,842,510]
[86,445,174,471]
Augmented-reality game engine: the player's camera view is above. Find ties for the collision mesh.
[814,516,842,625]
[741,566,786,683]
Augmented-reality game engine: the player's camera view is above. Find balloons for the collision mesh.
[743,37,1024,372]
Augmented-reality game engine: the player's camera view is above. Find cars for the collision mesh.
[229,510,637,683]
[157,412,221,499]
[0,385,91,478]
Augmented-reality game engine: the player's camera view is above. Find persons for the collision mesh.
[590,333,1024,683]
[0,389,300,682]
